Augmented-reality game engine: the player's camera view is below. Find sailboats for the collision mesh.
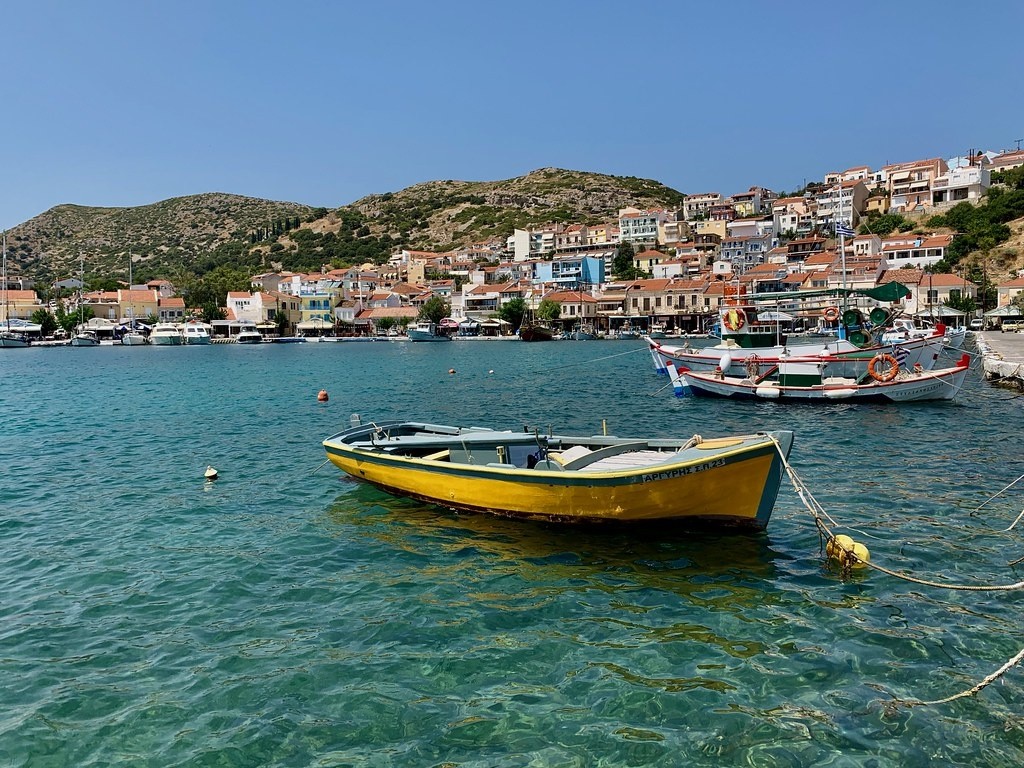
[518,267,553,342]
[122,246,149,346]
[69,250,101,346]
[0,235,31,347]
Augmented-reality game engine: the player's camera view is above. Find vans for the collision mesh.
[970,319,985,331]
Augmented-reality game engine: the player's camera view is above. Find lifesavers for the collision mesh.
[823,307,838,321]
[868,355,899,382]
[724,309,745,331]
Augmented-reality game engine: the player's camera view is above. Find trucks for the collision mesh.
[53,329,68,339]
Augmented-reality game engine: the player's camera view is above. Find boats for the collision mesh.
[570,329,594,340]
[640,325,970,403]
[322,419,795,533]
[615,320,641,340]
[706,257,967,351]
[649,324,666,340]
[551,332,567,341]
[407,322,453,342]
[272,337,306,343]
[174,319,212,345]
[236,323,263,345]
[146,321,183,346]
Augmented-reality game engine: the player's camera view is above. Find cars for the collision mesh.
[389,329,398,337]
[1000,319,1024,333]
[377,329,387,336]
